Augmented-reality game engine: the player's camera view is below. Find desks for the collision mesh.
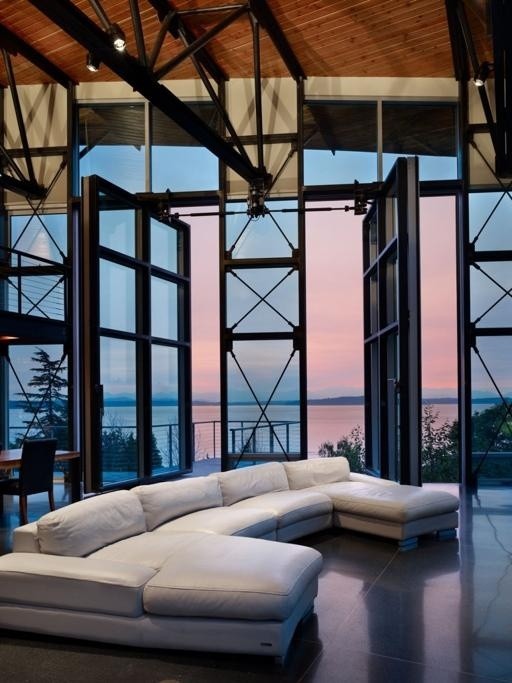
[0,455,460,665]
[0,448,80,503]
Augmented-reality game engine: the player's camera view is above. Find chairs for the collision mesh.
[0,439,57,526]
